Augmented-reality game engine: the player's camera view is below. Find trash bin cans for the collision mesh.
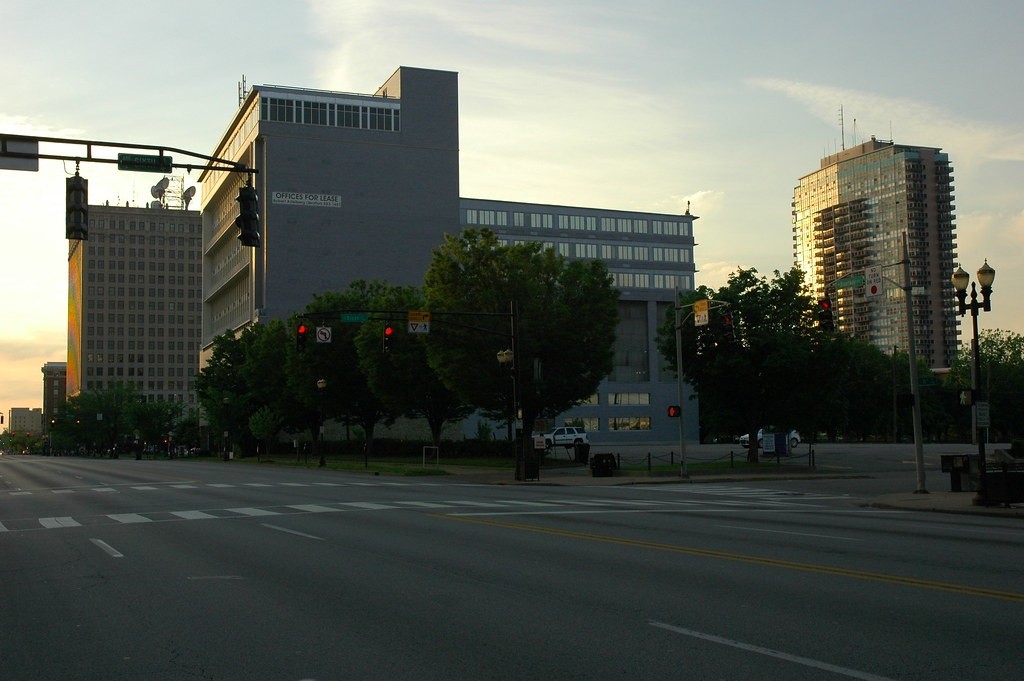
[136,452,142,460]
[941,454,954,472]
[516,437,540,482]
[592,453,617,477]
[574,444,590,465]
[950,468,970,492]
[224,450,229,461]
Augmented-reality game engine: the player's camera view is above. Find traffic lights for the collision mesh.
[669,405,681,417]
[76,418,80,424]
[721,312,735,345]
[164,436,168,444]
[51,419,55,426]
[957,388,976,406]
[295,325,308,353]
[817,297,834,335]
[383,325,396,357]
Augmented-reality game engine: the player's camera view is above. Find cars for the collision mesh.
[739,425,801,447]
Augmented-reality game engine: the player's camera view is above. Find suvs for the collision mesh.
[531,426,590,449]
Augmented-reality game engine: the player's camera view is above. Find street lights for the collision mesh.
[497,345,515,456]
[221,397,232,462]
[317,377,326,466]
[949,256,996,505]
